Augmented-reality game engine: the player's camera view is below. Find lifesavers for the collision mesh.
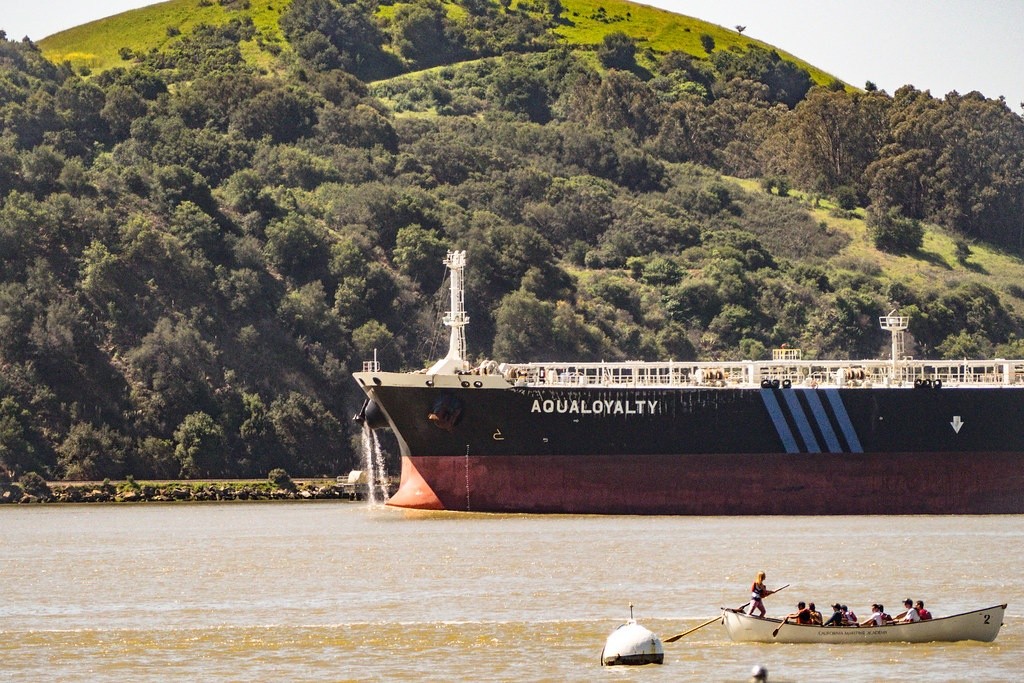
[783,379,792,388]
[923,379,932,388]
[761,380,771,388]
[933,379,942,388]
[914,378,923,388]
[771,379,780,387]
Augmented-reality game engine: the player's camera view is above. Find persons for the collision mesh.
[785,601,823,625]
[902,598,932,623]
[748,571,774,618]
[824,604,857,627]
[858,603,892,626]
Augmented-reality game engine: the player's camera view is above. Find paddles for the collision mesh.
[663,584,790,643]
[772,614,790,638]
[893,612,907,620]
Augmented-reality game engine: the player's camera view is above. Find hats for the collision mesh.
[902,599,912,605]
[831,604,840,610]
[796,602,805,606]
[841,605,847,611]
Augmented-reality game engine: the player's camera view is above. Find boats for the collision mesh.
[351,251,1024,516]
[721,604,1006,644]
